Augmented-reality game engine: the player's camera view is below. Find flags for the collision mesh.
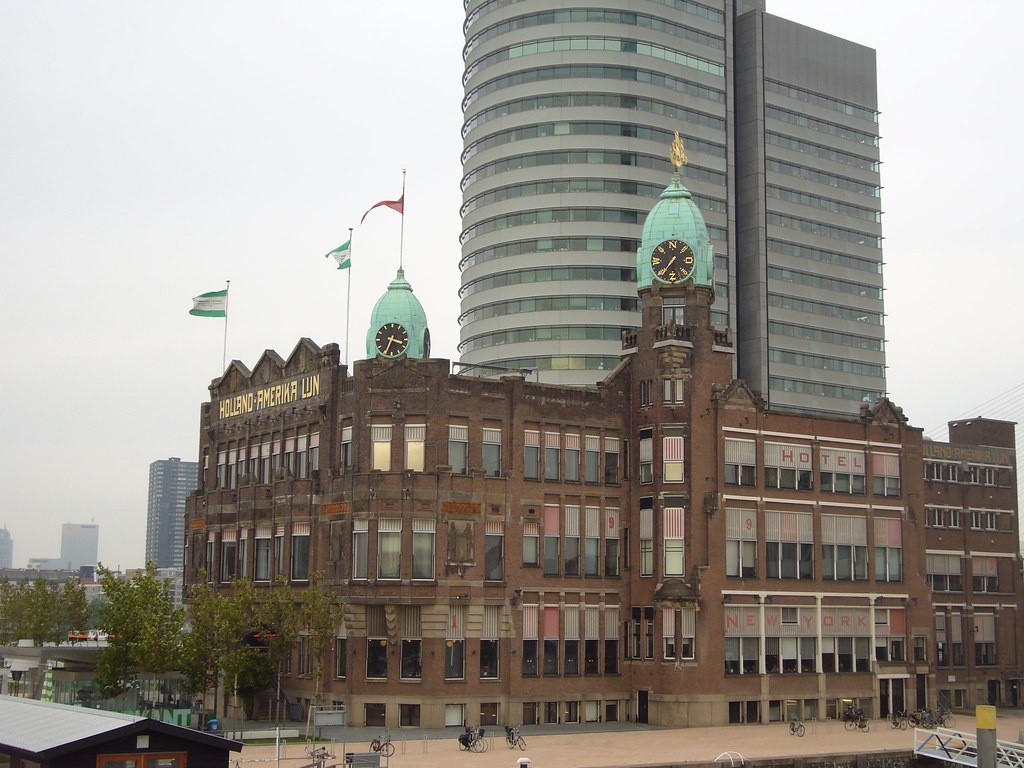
[189,289,226,317]
[325,240,351,269]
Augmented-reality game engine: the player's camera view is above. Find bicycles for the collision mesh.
[369,731,395,757]
[506,725,527,752]
[458,726,488,753]
[844,702,870,732]
[788,716,805,737]
[890,701,955,731]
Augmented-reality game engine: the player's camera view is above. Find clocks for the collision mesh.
[376,322,409,358]
[650,239,695,285]
